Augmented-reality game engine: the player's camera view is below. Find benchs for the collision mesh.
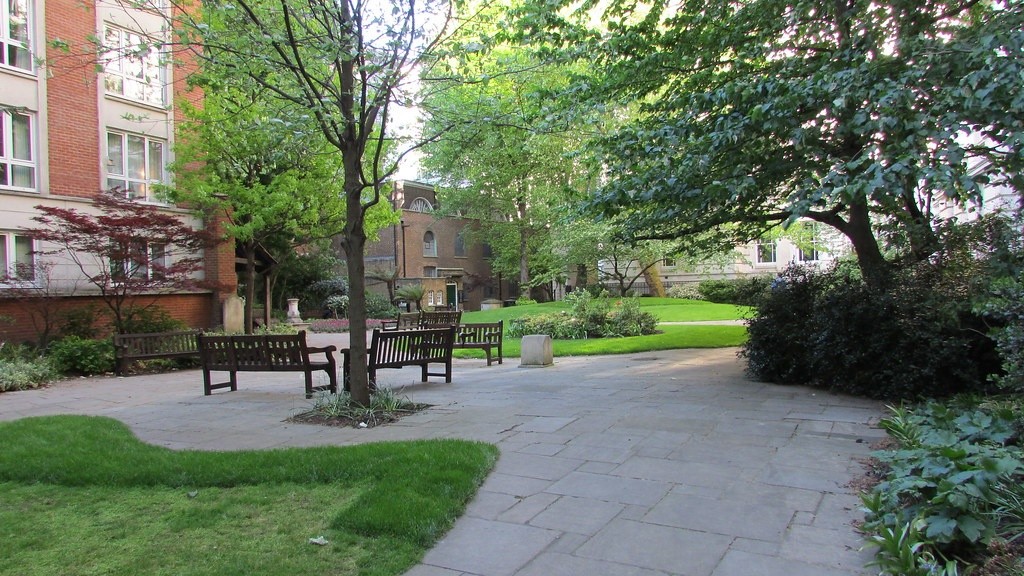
[113,328,206,377]
[420,309,462,332]
[421,320,504,367]
[340,324,457,390]
[196,330,337,399]
[381,310,421,341]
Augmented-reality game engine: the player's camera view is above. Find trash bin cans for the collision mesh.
[504,299,516,307]
